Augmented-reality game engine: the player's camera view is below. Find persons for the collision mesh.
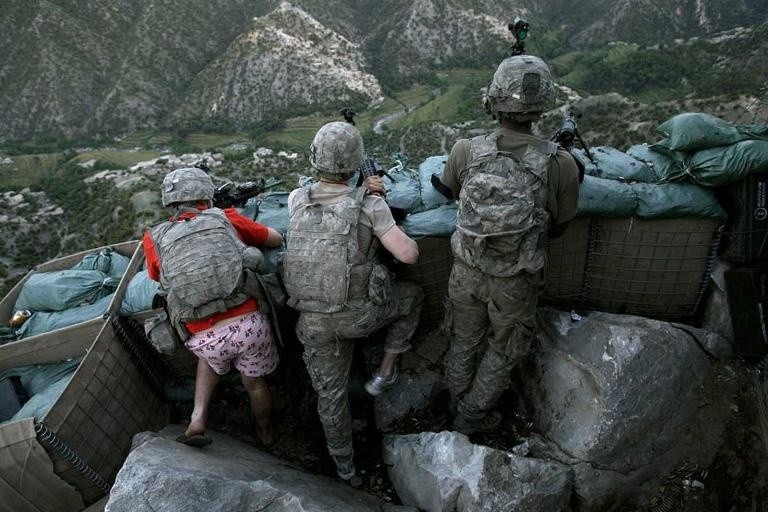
[285,121,427,491]
[441,52,580,436]
[141,167,285,447]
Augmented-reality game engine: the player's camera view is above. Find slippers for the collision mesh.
[174,433,213,448]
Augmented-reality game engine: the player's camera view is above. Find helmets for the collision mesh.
[486,55,557,123]
[161,167,215,209]
[308,121,365,175]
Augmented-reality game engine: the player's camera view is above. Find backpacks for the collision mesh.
[275,184,396,314]
[149,207,285,325]
[450,132,560,279]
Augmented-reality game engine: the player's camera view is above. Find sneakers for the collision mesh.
[331,448,356,480]
[449,400,502,439]
[364,364,400,396]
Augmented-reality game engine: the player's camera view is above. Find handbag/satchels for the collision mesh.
[144,312,182,358]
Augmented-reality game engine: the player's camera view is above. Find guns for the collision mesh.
[551,115,597,164]
[339,107,407,272]
[150,177,285,228]
